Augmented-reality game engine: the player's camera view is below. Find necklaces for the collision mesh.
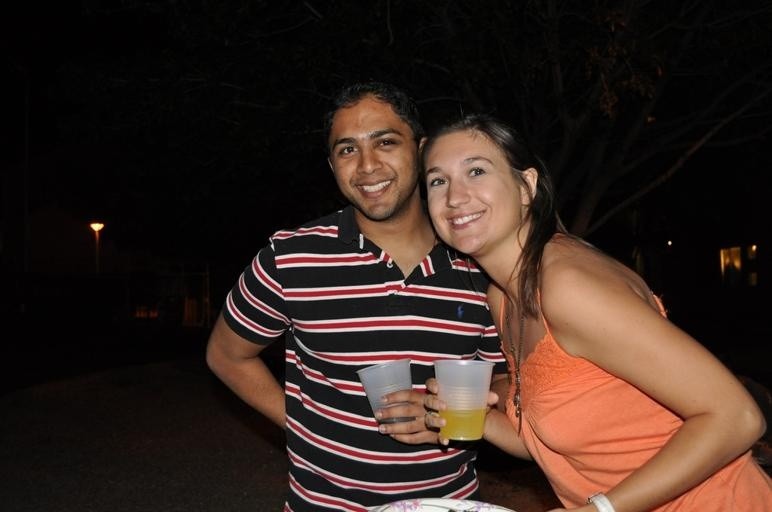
[506,298,524,438]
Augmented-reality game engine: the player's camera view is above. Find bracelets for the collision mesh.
[587,493,616,512]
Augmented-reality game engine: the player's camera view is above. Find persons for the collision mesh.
[421,115,772,512]
[206,81,510,512]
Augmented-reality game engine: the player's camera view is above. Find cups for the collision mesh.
[355,358,415,426]
[434,358,496,442]
